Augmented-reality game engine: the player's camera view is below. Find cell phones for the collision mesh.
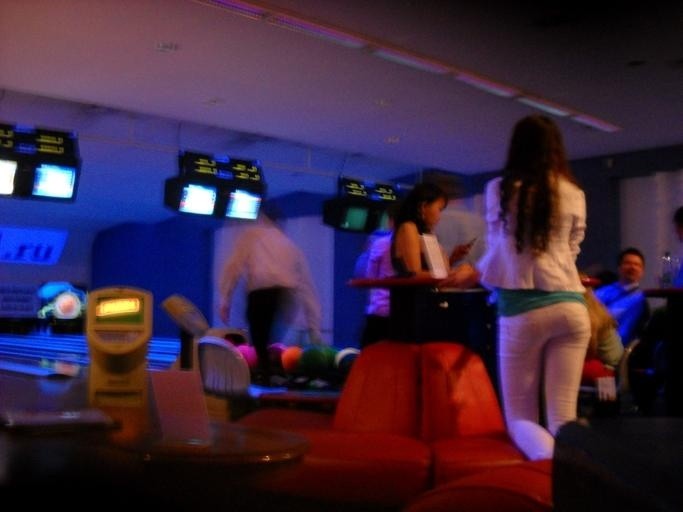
[466,238,477,247]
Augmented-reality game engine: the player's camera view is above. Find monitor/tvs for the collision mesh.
[217,185,265,222]
[0,157,24,199]
[373,201,396,235]
[24,159,80,202]
[164,177,220,219]
[323,195,380,235]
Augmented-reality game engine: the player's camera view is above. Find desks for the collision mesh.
[349,275,599,405]
[641,287,683,333]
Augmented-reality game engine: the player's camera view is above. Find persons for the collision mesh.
[358,202,402,350]
[438,252,491,289]
[591,247,648,348]
[580,286,625,419]
[480,114,594,461]
[216,200,324,383]
[390,183,480,289]
[668,206,682,287]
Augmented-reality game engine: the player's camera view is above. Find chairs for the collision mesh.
[0,310,682,512]
